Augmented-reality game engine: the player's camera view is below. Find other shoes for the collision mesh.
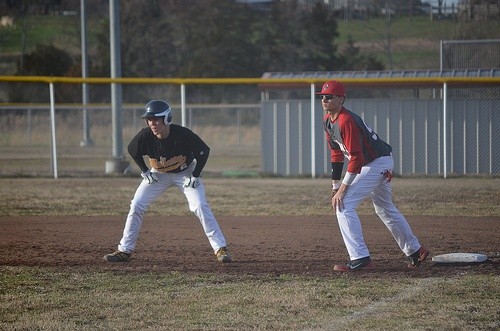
[104,250,132,262]
[217,247,231,263]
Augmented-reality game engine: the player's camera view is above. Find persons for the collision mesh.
[314,81,431,272]
[103,100,235,262]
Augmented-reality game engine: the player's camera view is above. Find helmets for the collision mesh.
[141,100,173,126]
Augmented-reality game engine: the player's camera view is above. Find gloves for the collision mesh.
[140,168,159,184]
[184,174,200,188]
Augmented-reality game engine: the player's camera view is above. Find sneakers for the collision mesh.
[405,247,430,268]
[332,255,376,271]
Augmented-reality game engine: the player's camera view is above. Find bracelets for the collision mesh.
[332,182,340,189]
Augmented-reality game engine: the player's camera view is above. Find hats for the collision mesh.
[314,80,346,96]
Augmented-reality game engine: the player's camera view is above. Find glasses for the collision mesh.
[320,95,336,101]
